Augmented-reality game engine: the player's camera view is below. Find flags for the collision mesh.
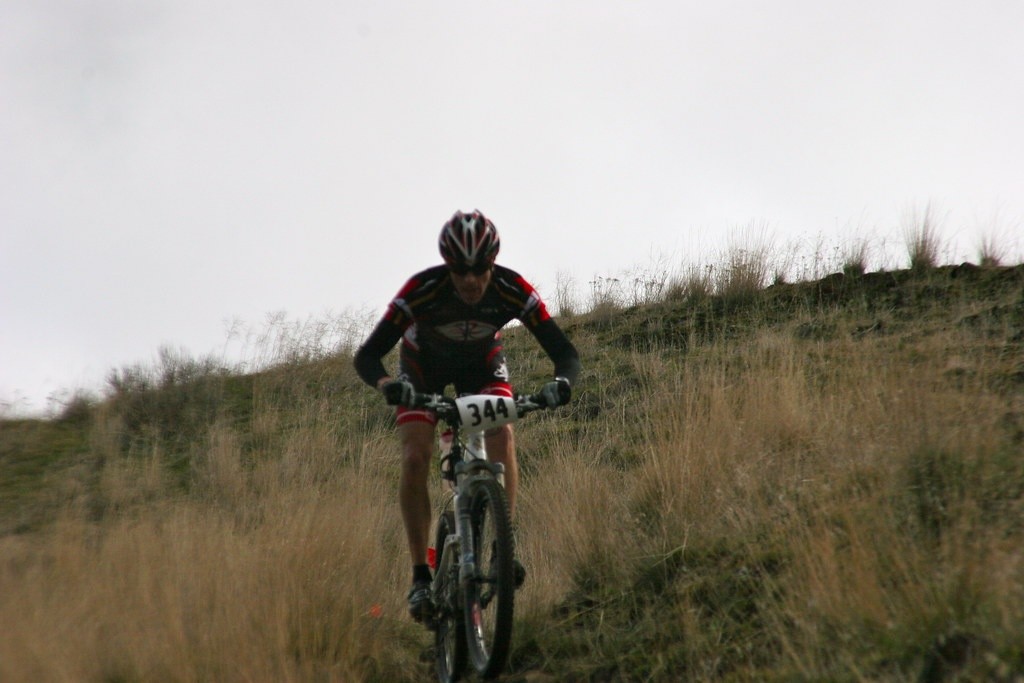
[427,548,436,569]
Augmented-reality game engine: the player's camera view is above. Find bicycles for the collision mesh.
[413,389,544,683]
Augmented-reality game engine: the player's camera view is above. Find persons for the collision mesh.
[353,208,579,623]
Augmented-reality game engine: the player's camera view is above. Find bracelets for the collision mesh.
[555,376,569,386]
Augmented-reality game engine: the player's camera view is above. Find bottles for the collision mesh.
[438,427,453,472]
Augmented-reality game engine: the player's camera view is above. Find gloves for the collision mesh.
[537,380,572,412]
[382,379,416,410]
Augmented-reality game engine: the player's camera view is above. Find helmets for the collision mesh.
[437,207,501,263]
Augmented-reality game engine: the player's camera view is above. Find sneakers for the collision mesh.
[490,539,526,585]
[405,580,436,615]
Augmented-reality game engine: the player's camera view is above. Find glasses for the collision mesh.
[450,262,487,277]
